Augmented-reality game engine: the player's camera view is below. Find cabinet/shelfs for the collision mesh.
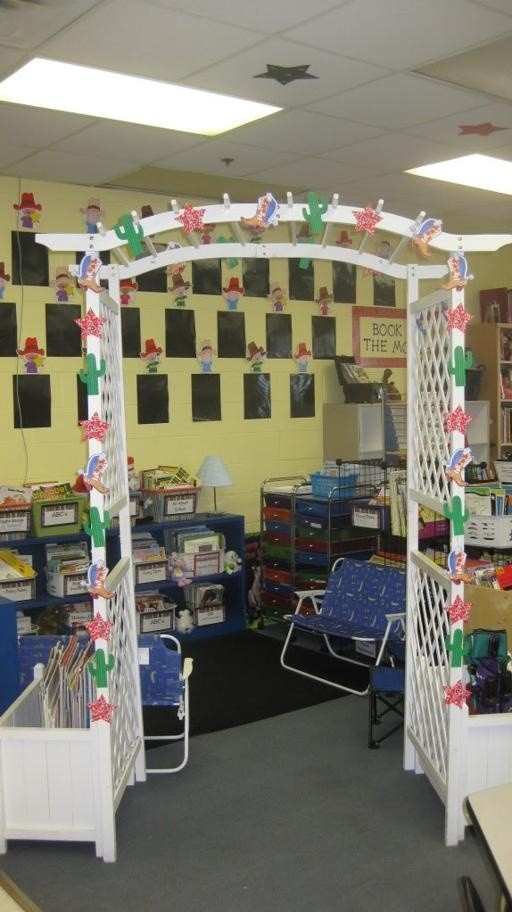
[464,321,512,461]
[1,511,250,653]
[263,488,379,633]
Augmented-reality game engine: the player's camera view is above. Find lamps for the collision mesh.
[193,454,231,518]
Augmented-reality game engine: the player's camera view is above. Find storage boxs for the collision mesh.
[1,494,85,539]
[140,486,197,522]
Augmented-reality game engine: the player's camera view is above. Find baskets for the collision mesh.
[464,514,512,549]
[310,470,358,499]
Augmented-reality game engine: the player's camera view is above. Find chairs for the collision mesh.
[137,632,191,776]
[280,556,407,722]
